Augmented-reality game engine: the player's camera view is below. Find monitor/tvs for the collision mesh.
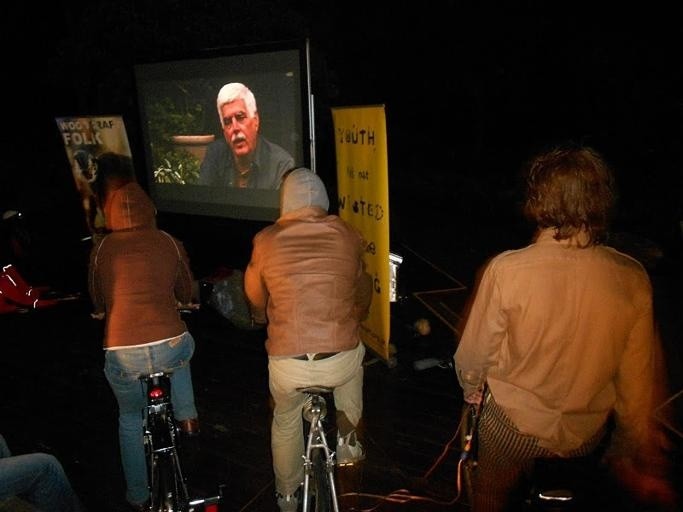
[132,34,310,223]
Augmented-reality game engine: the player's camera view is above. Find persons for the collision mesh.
[246,169,370,512]
[0,210,58,314]
[197,83,294,187]
[455,150,672,506]
[0,436,75,512]
[86,179,198,512]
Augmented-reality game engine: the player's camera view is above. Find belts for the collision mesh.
[292,351,340,361]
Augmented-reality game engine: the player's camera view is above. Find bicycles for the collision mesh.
[138,371,225,512]
[448,357,576,512]
[297,384,339,512]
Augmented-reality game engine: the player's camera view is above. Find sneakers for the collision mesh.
[181,419,201,432]
[336,441,366,467]
[276,491,302,512]
[136,504,149,512]
